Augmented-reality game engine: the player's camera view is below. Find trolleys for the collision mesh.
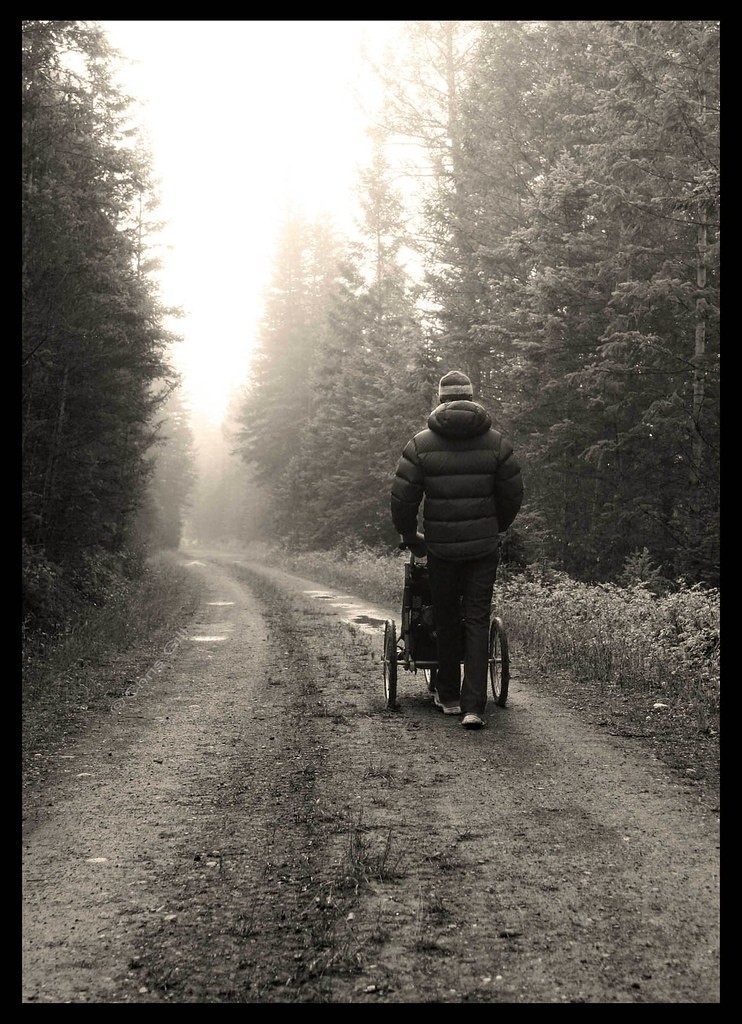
[377,533,512,713]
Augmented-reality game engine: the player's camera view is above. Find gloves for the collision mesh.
[404,533,429,557]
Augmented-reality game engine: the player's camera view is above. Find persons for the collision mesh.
[390,371,525,730]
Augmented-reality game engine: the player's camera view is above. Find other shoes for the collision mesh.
[434,692,462,714]
[462,712,485,727]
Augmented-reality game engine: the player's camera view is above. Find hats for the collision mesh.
[438,370,473,404]
